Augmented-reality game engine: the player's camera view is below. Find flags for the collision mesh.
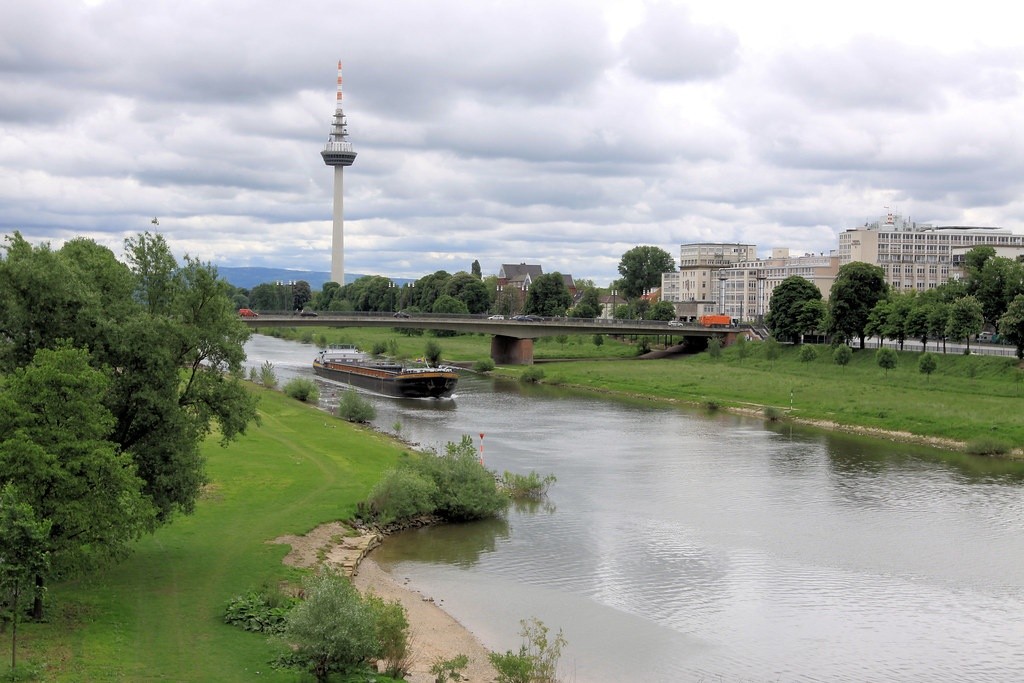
[416,355,427,363]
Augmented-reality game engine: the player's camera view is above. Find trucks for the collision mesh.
[699,315,740,329]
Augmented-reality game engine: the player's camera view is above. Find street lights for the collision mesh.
[408,281,415,311]
[521,284,529,316]
[496,285,503,314]
[611,289,618,319]
[388,281,395,312]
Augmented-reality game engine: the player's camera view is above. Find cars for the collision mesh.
[294,308,318,317]
[393,313,412,319]
[509,314,545,323]
[239,309,259,317]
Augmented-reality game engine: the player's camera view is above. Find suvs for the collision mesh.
[487,314,505,320]
[668,321,684,327]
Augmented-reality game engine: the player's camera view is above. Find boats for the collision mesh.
[313,345,459,400]
